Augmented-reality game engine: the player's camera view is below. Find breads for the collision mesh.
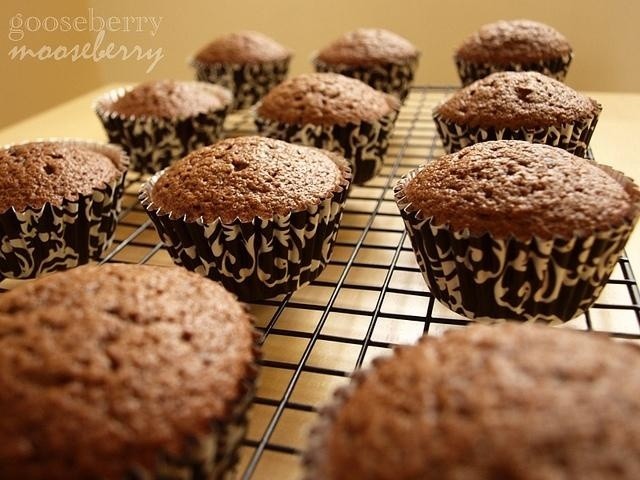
[393,139,640,324]
[0,139,131,280]
[95,20,603,185]
[302,321,640,480]
[0,264,264,480]
[139,135,352,299]
